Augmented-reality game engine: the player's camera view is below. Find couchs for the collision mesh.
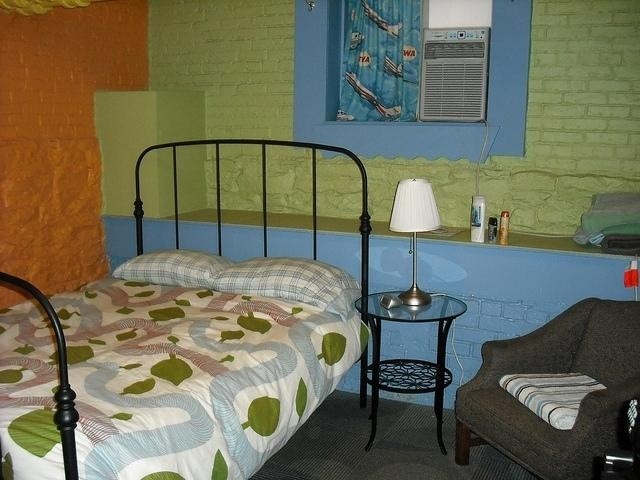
[454,298,640,480]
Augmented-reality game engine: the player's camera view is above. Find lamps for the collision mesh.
[389,178,466,387]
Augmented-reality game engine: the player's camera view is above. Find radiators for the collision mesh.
[420,26,492,196]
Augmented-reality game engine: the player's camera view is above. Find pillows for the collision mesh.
[208,256,363,321]
[112,246,227,289]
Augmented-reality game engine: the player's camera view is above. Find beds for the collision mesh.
[0,137,372,480]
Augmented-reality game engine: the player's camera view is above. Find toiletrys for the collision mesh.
[470,194,510,245]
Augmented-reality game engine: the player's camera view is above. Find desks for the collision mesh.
[354,292,468,456]
